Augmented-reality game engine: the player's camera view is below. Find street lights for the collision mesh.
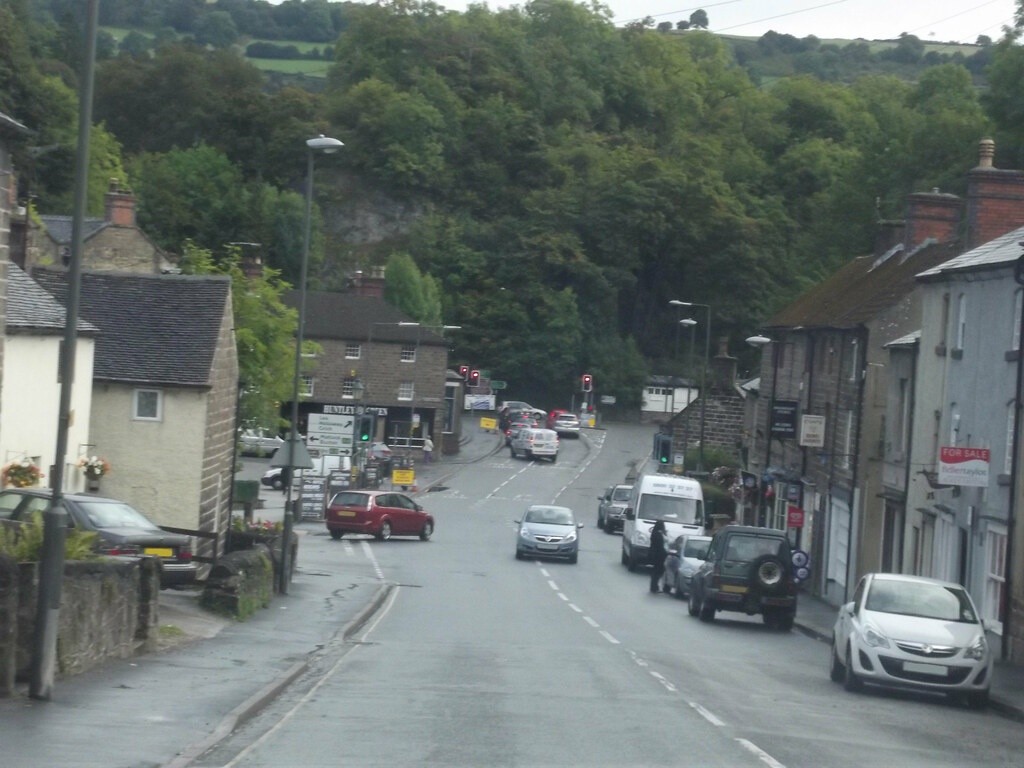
[349,378,365,466]
[277,132,347,596]
[743,335,780,527]
[675,317,698,474]
[362,320,420,350]
[668,299,712,479]
[405,324,461,470]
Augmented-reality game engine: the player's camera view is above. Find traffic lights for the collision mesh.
[459,365,470,382]
[359,414,374,442]
[652,433,674,466]
[582,374,593,393]
[471,370,481,389]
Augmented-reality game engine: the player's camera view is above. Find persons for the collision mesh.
[422,435,434,463]
[649,520,668,593]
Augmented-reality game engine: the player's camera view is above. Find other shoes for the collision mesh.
[651,589,662,593]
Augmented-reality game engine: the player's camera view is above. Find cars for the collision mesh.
[498,406,539,446]
[324,490,435,542]
[661,534,737,606]
[545,409,568,428]
[0,488,198,589]
[495,401,547,421]
[238,429,285,456]
[552,413,581,438]
[597,482,633,534]
[354,440,393,463]
[514,505,583,565]
[829,572,993,704]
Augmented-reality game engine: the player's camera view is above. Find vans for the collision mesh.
[509,428,560,463]
[621,472,714,571]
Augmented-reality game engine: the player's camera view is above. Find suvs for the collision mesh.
[687,524,798,631]
